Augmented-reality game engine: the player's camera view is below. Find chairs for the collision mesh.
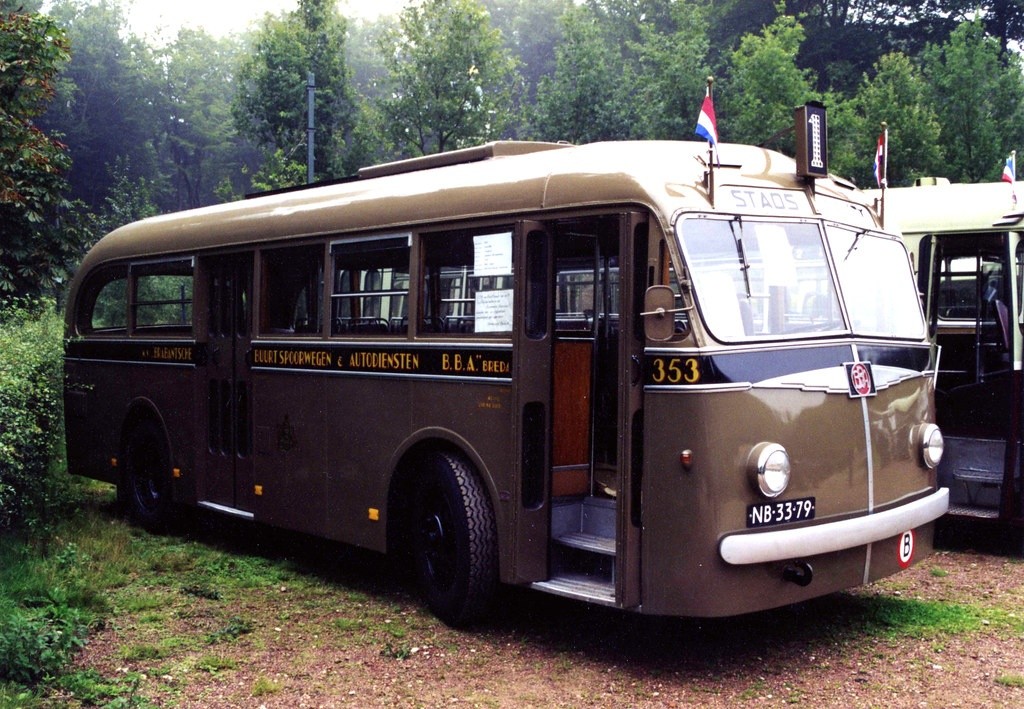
[275,314,473,333]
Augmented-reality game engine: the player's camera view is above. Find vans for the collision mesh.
[63,99,951,637]
[578,177,1023,559]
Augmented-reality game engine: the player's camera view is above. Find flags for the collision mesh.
[873,133,884,188]
[1002,155,1017,203]
[695,86,721,170]
